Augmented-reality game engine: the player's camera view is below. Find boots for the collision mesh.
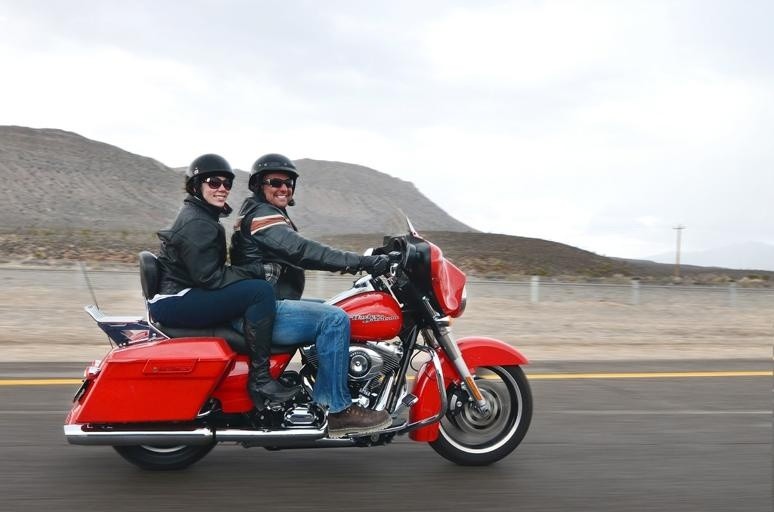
[245,318,301,411]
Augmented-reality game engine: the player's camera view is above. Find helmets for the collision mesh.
[185,153,235,185]
[248,154,299,193]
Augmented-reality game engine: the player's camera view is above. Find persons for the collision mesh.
[148,154,302,411]
[230,154,393,437]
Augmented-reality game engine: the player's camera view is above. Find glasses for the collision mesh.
[265,178,295,188]
[205,175,233,191]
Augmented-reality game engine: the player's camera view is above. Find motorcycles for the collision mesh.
[61,209,531,472]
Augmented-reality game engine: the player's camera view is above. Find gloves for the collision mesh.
[363,254,390,276]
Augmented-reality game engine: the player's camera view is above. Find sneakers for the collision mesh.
[327,405,393,439]
